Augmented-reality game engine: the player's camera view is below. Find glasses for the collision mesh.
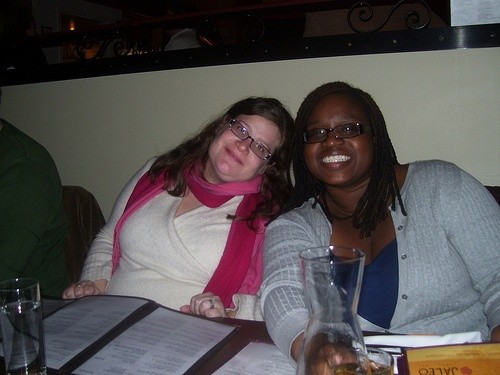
[229,117,271,160]
[302,122,373,144]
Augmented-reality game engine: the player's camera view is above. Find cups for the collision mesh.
[347,346,394,375]
[0,278,47,375]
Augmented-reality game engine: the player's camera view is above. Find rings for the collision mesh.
[209,300,214,309]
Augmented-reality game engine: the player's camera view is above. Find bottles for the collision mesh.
[299,245,373,375]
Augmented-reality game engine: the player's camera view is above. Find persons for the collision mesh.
[62,97,295,321]
[0,118,71,298]
[258,82,500,375]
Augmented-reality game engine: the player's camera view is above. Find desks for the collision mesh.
[0,312,276,375]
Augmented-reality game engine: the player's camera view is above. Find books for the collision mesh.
[42,295,238,375]
